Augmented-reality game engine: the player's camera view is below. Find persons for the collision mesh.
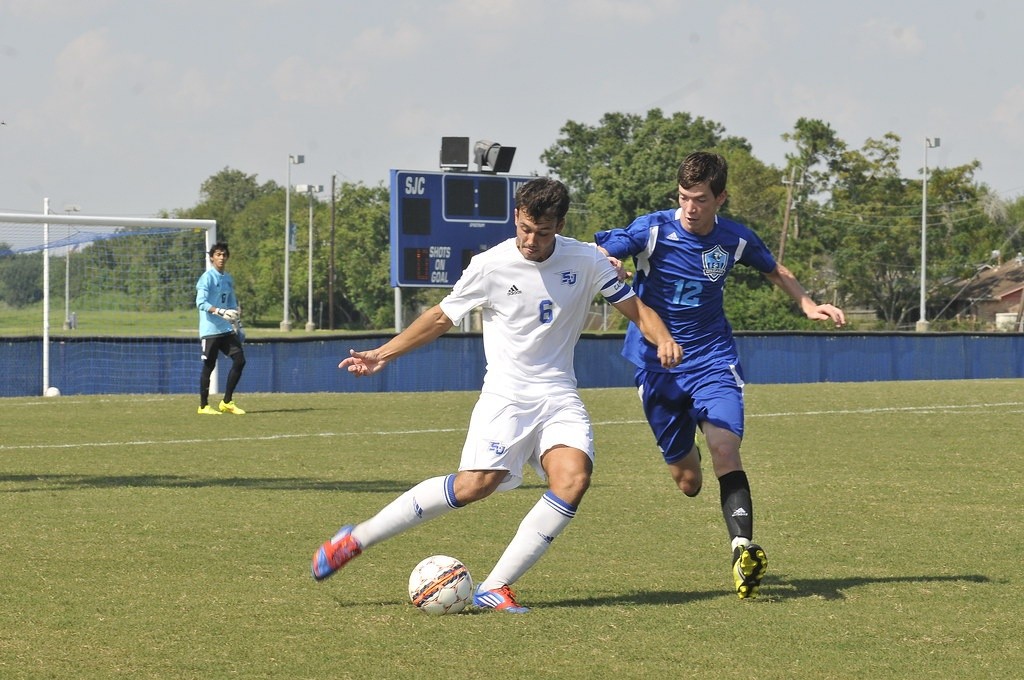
[592,152,845,600]
[312,178,684,613]
[196,243,247,415]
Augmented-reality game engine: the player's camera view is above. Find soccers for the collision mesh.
[408,554,473,616]
[46,386,60,397]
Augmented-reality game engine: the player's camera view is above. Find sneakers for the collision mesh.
[198,405,222,414]
[732,544,768,599]
[312,524,362,580]
[218,400,245,414]
[472,582,531,615]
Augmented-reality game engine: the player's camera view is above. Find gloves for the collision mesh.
[212,308,240,321]
